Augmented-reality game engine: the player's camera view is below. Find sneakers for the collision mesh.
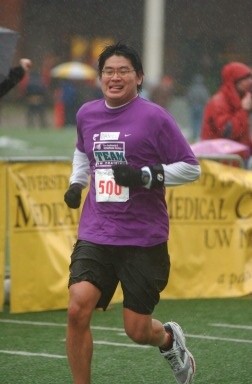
[159,322,196,384]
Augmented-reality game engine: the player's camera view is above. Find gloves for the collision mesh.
[111,164,150,188]
[64,183,83,208]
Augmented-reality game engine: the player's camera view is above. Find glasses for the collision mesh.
[102,68,137,77]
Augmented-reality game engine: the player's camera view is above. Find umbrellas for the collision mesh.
[47,60,99,83]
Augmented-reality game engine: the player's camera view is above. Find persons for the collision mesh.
[0,46,213,144]
[202,61,252,169]
[64,42,196,384]
